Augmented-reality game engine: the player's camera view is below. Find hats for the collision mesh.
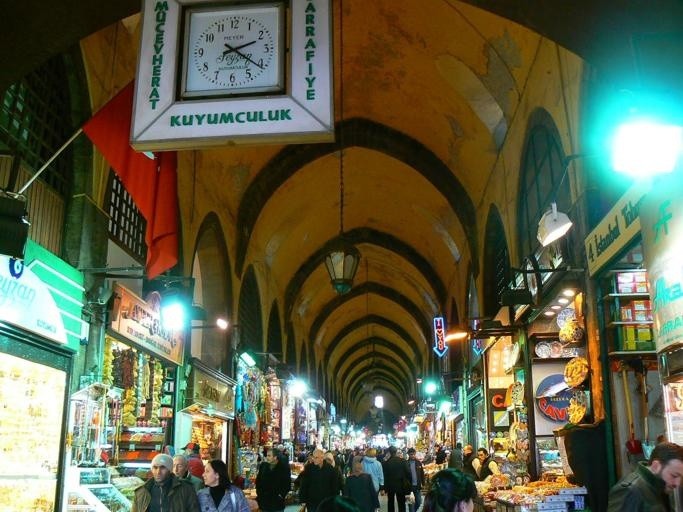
[150,453,174,476]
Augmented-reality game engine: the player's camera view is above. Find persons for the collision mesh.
[295,445,501,512]
[133,443,249,511]
[609,440,682,511]
[256,444,291,512]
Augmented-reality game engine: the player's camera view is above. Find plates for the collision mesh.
[550,340,563,358]
[510,342,520,365]
[556,308,576,329]
[510,381,524,405]
[534,342,552,358]
[566,391,587,423]
[562,357,589,388]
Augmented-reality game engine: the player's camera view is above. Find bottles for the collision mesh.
[80,471,104,483]
[97,495,120,512]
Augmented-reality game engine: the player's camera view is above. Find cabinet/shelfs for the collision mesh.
[600,265,656,360]
[134,360,175,421]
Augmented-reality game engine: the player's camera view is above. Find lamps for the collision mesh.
[321,1,361,295]
[534,151,595,247]
[359,252,380,417]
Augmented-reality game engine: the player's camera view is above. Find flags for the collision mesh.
[82,77,179,281]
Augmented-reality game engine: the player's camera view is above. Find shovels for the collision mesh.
[621,369,643,454]
[641,368,656,460]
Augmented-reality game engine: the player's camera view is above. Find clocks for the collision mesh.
[174,3,289,99]
